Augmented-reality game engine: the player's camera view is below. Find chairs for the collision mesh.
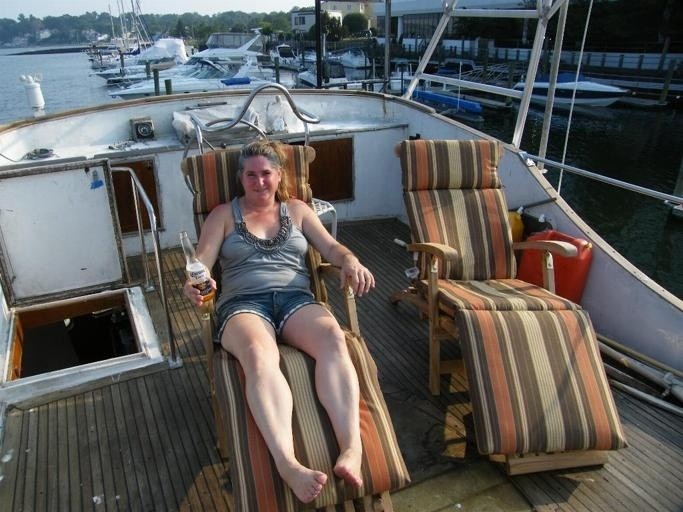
[390,137,630,477]
[180,145,411,510]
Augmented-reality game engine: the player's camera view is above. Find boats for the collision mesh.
[0,86,680,508]
[511,73,632,107]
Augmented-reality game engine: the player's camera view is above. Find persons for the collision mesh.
[183,138,375,504]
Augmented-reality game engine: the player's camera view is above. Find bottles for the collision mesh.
[179,231,215,302]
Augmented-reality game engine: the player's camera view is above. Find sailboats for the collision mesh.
[87,5,154,53]
